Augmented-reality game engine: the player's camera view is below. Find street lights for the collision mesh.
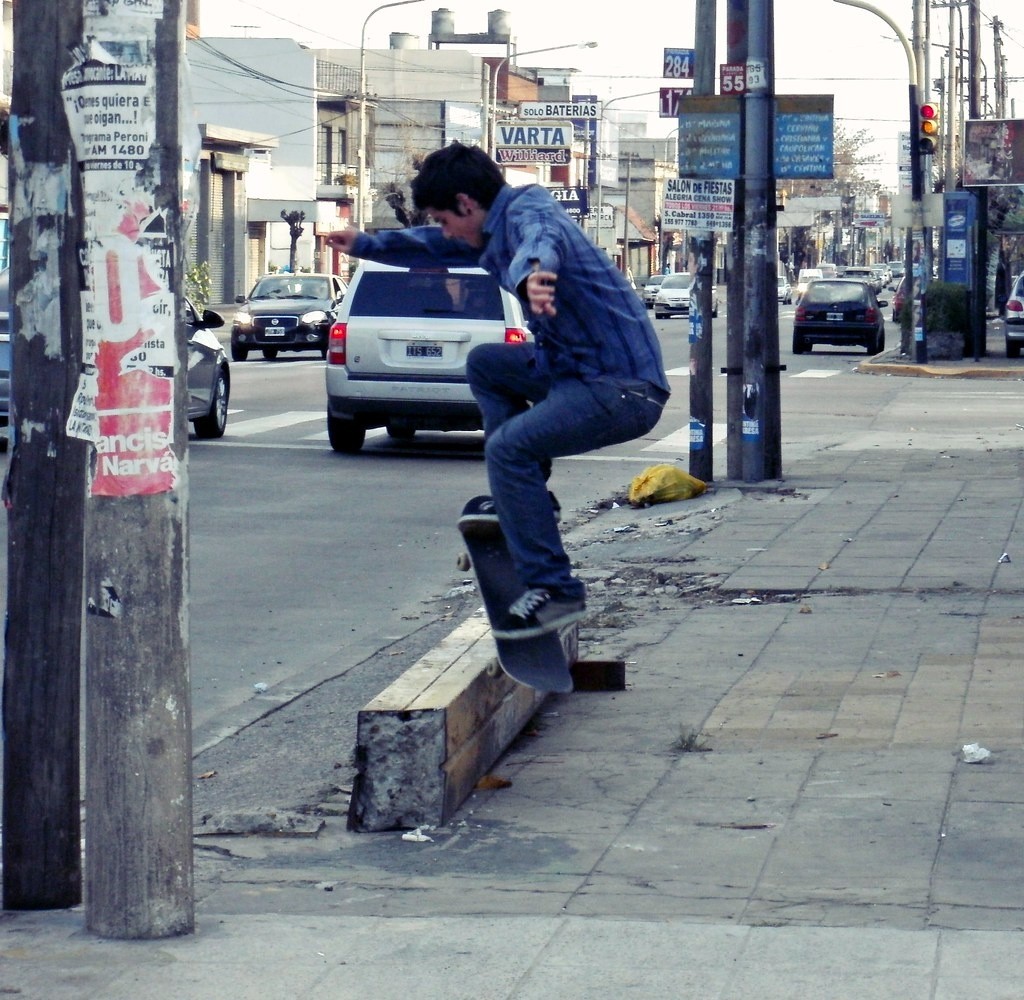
[490,41,598,163]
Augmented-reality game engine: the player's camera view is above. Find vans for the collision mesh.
[797,267,824,302]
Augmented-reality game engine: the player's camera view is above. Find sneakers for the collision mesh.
[495,577,588,638]
[458,492,562,534]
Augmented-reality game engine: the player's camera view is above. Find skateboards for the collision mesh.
[455,494,574,697]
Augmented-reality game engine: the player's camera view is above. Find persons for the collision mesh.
[664,263,671,276]
[324,143,671,640]
[627,265,633,280]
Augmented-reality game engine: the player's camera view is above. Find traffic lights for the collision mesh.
[920,101,939,152]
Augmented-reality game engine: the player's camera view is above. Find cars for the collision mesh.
[653,272,718,320]
[888,275,920,323]
[815,261,905,294]
[776,275,792,305]
[0,265,230,440]
[641,274,666,309]
[792,278,888,355]
[231,272,349,361]
[994,271,1024,358]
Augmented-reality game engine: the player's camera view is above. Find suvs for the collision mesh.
[324,261,537,455]
